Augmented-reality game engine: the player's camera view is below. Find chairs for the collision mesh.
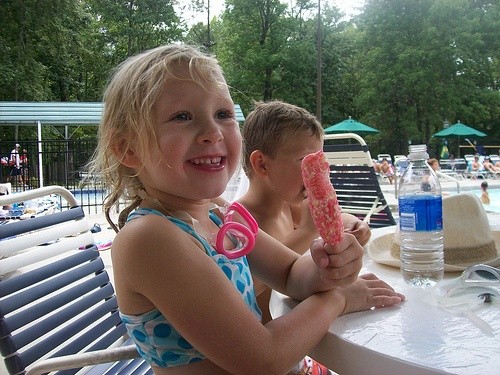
[322,132,410,229]
[440,154,500,182]
[0,186,153,375]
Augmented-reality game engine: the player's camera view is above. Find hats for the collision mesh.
[367,194,500,272]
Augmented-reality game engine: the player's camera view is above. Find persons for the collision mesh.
[7,144,27,187]
[229,100,371,325]
[19,149,28,186]
[372,154,500,204]
[86,46,406,375]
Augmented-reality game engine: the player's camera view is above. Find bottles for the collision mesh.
[399,143,444,290]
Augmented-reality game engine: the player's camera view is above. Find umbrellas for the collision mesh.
[433,120,487,158]
[325,116,379,144]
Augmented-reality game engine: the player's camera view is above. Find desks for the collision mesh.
[267,222,500,375]
